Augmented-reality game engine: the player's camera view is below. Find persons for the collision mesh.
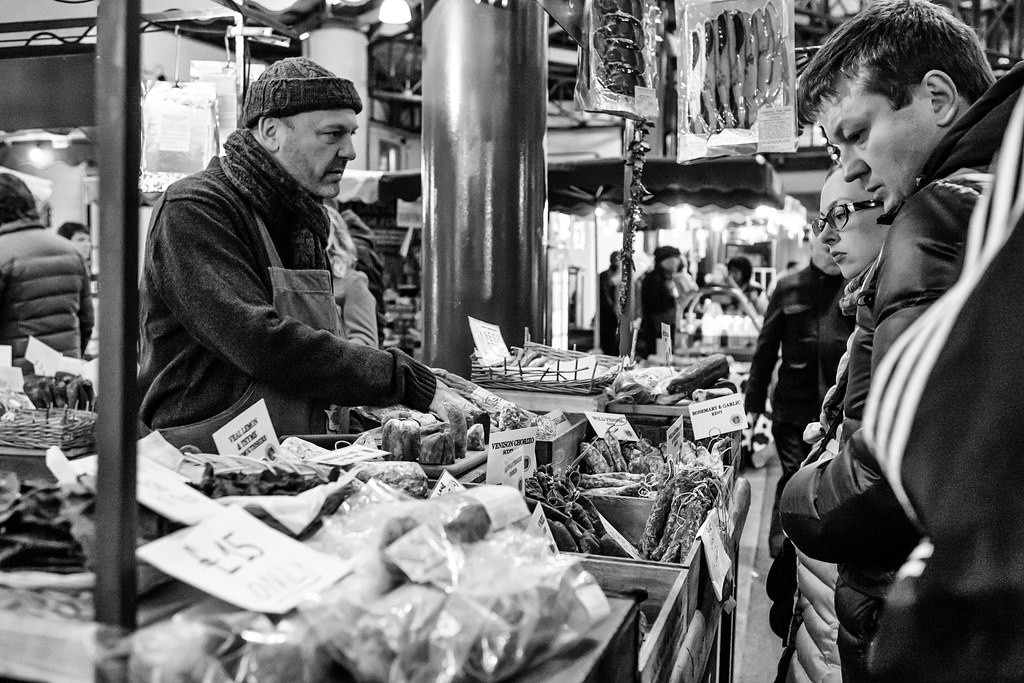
[738,229,864,472]
[567,245,772,363]
[783,165,899,683]
[136,54,477,457]
[780,1,1023,683]
[0,173,95,377]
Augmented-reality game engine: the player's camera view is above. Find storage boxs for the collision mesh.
[0,320,750,683]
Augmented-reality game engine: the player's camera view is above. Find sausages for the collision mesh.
[516,434,725,563]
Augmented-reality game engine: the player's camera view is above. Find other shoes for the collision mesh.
[768,533,785,557]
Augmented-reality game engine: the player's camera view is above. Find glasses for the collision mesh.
[811,199,885,237]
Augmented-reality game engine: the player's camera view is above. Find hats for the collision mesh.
[654,246,680,258]
[243,57,363,127]
